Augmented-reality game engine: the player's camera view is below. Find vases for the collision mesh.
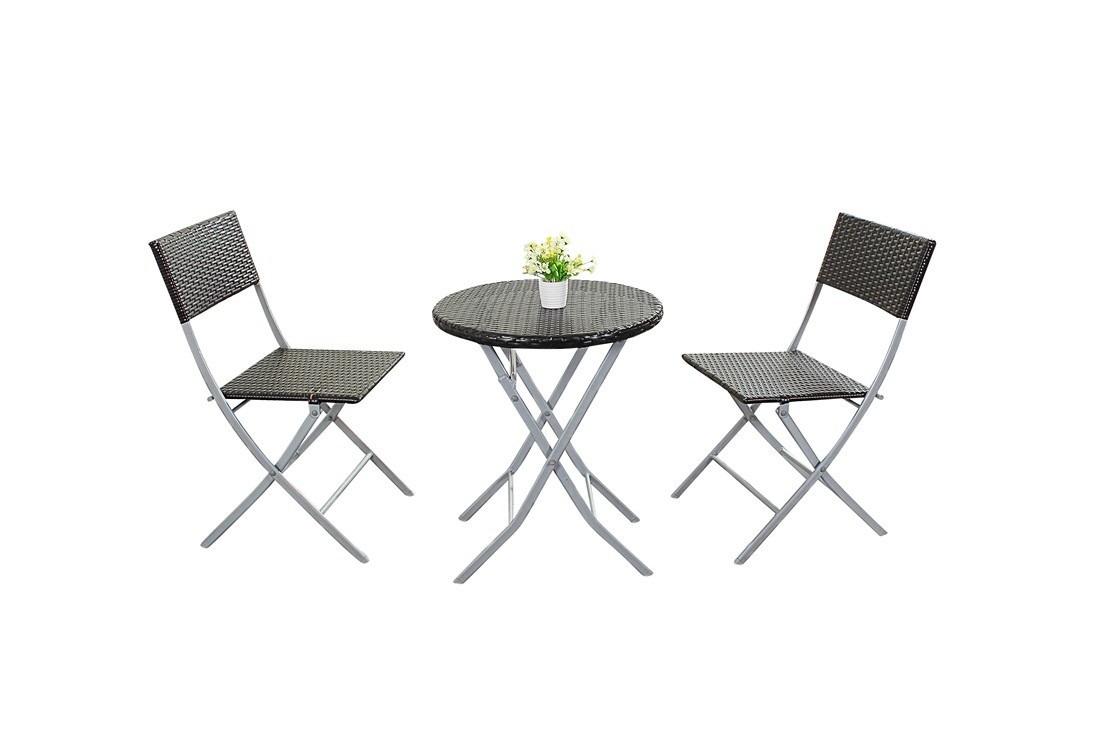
[539,280,568,309]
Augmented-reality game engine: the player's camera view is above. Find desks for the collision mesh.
[433,280,651,582]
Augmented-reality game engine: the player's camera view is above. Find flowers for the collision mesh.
[524,235,595,282]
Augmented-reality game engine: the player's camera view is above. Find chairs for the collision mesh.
[672,212,934,566]
[148,209,415,564]
